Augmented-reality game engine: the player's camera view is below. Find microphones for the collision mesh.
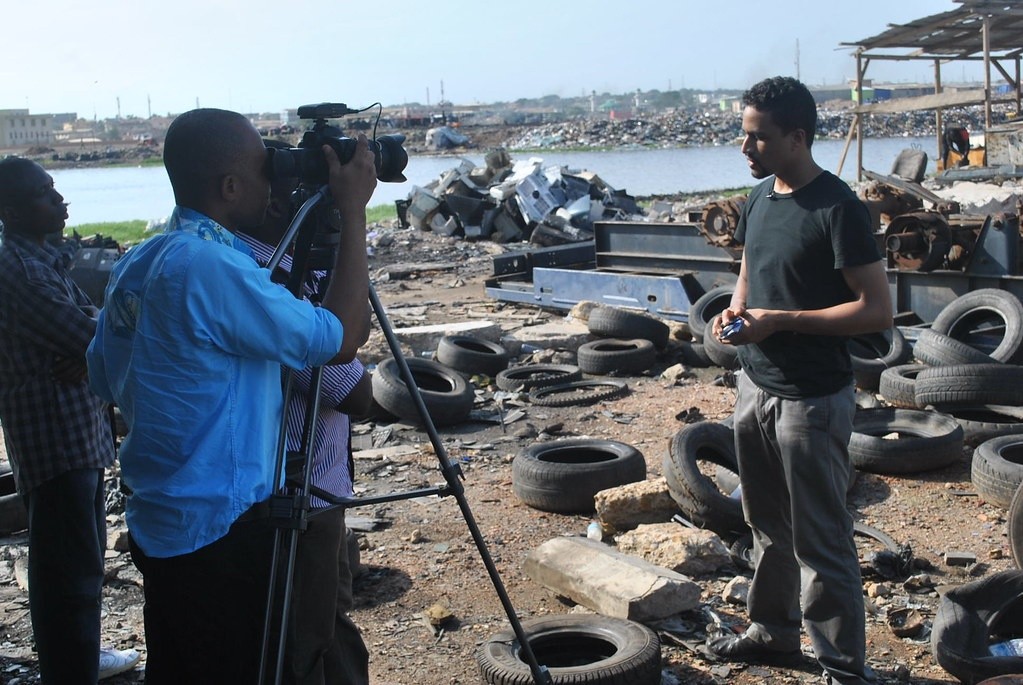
[766,192,773,198]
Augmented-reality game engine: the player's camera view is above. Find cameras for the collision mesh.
[266,103,407,183]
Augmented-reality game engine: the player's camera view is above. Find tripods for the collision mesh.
[260,187,549,685]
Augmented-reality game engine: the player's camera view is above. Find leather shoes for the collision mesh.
[706,632,802,665]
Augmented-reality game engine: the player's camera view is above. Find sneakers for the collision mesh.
[98,646,141,680]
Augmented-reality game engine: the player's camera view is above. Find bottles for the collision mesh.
[587,520,602,543]
[987,638,1023,657]
[520,344,539,354]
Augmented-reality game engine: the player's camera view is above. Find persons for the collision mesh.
[1,146,145,684]
[84,105,380,685]
[224,130,385,685]
[711,75,894,685]
